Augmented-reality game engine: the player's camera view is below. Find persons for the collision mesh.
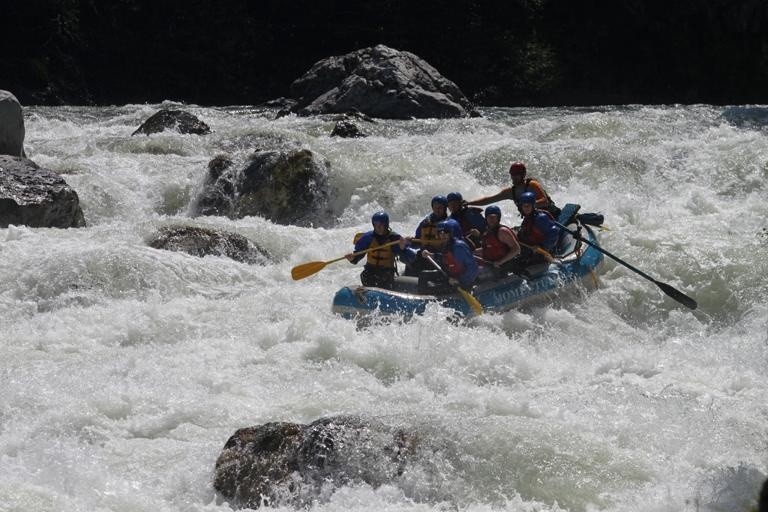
[474,204,521,271]
[344,210,416,291]
[461,161,561,222]
[416,219,477,295]
[404,194,449,277]
[511,191,560,269]
[446,191,486,253]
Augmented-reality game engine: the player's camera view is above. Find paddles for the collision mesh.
[291,239,410,281]
[519,241,553,260]
[555,221,697,310]
[354,232,435,244]
[425,254,482,315]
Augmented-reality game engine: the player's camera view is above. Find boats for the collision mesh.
[330,221,607,329]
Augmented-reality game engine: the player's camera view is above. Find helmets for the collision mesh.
[518,191,536,209]
[437,218,462,239]
[509,162,526,175]
[485,205,501,218]
[447,191,463,202]
[372,211,389,226]
[431,194,448,209]
